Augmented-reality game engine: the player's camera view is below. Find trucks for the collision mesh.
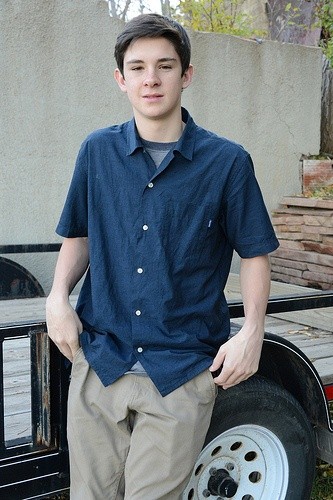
[0,243,331,499]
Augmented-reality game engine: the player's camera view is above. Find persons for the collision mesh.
[46,11,281,500]
